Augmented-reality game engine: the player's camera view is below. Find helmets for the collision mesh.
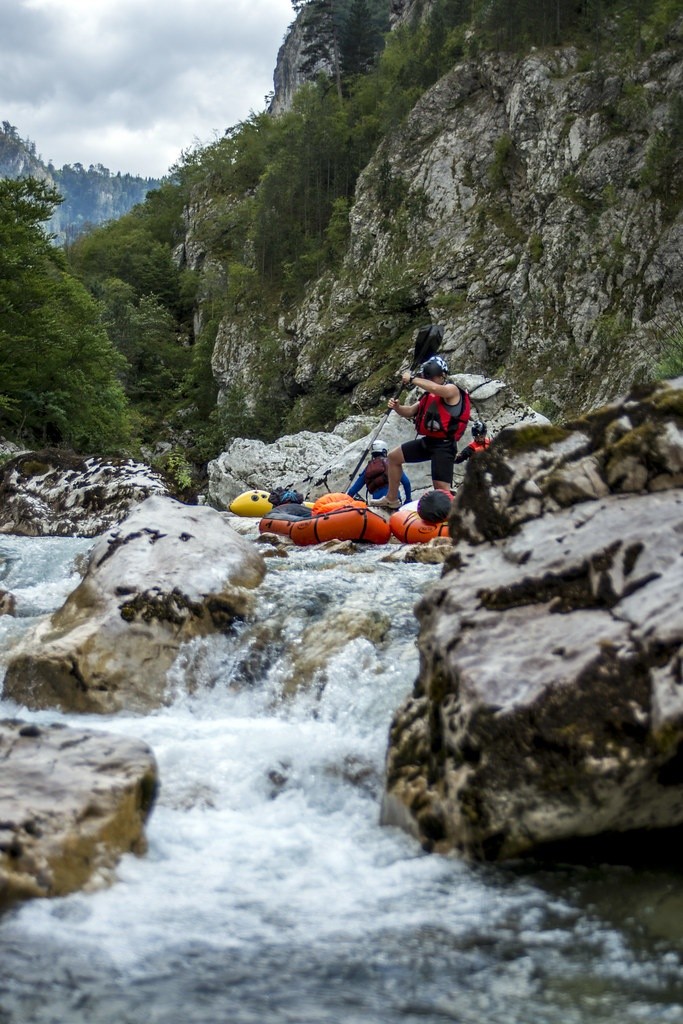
[471,422,486,436]
[371,440,389,452]
[422,357,448,374]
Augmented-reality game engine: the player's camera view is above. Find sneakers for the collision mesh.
[370,496,402,508]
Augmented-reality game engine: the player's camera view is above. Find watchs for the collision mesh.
[409,375,416,384]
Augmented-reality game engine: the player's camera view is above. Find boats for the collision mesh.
[229,487,316,518]
[259,503,391,547]
[390,498,451,545]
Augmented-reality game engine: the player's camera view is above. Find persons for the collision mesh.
[455,421,493,466]
[348,439,412,513]
[371,356,471,509]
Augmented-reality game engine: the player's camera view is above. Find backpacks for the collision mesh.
[364,458,391,492]
